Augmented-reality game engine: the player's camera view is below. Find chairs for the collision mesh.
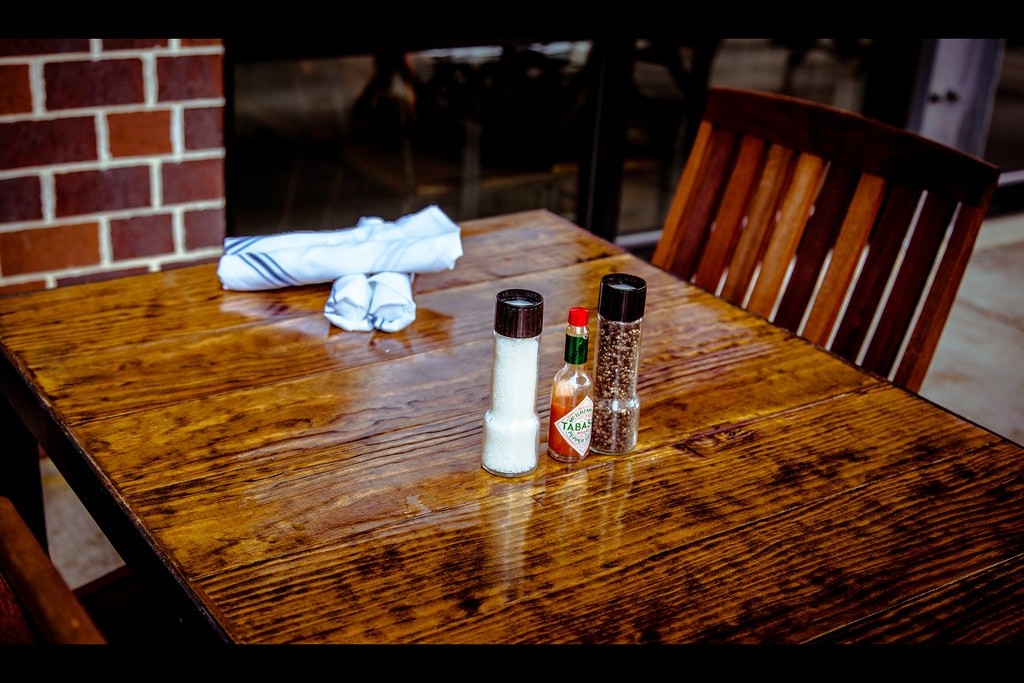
[649,82,1003,393]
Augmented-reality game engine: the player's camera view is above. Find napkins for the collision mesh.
[215,204,465,331]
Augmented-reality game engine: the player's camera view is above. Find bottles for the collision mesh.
[481,288,544,478]
[590,272,647,456]
[547,306,594,463]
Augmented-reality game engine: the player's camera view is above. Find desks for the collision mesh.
[0,209,1024,648]
